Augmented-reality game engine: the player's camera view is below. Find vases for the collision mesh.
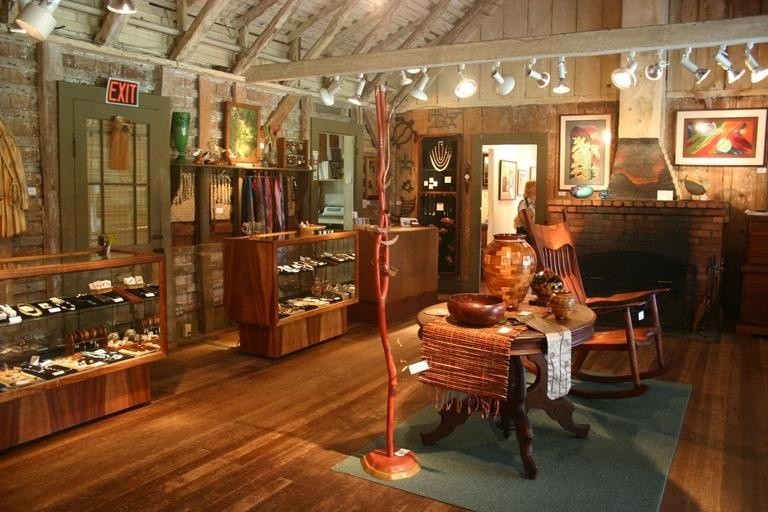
[172,111,191,164]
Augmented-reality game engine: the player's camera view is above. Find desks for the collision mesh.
[416,299,595,482]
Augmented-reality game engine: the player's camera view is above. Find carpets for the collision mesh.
[328,364,693,512]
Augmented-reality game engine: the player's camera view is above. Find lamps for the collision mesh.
[19,0,62,42]
[608,39,768,92]
[107,0,136,15]
[315,43,572,111]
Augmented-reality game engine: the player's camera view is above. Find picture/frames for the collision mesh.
[675,108,768,168]
[224,98,260,166]
[529,166,537,181]
[499,160,517,201]
[517,168,528,196]
[481,153,491,186]
[365,155,380,200]
[557,115,614,193]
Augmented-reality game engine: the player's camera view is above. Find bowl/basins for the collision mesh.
[447,291,506,328]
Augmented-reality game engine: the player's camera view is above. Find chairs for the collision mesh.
[524,207,671,405]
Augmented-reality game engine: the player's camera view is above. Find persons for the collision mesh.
[512,179,536,247]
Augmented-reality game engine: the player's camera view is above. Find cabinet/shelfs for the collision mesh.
[219,226,361,362]
[417,130,465,282]
[735,209,767,335]
[0,247,171,453]
[311,118,364,229]
